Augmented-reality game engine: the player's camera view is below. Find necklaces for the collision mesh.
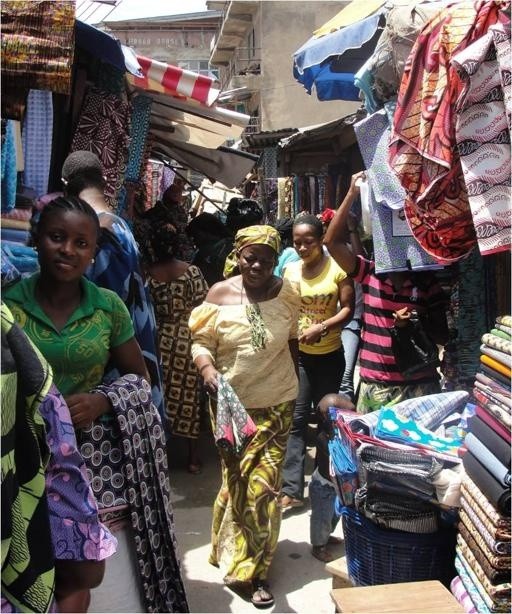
[241,277,271,305]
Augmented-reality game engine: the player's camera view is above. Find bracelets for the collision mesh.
[199,364,213,376]
[320,322,330,337]
[348,229,359,234]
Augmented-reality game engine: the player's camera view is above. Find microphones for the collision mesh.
[325,555,353,588]
[330,579,468,614]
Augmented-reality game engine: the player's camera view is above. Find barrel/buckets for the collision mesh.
[250,579,274,607]
[311,548,333,562]
[189,457,201,474]
[327,536,342,544]
[280,495,304,509]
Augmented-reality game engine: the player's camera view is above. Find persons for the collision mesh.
[315,208,369,402]
[308,393,357,563]
[61,150,170,442]
[323,170,451,416]
[140,224,210,474]
[3,196,150,612]
[188,225,301,606]
[282,213,356,508]
[123,177,301,288]
